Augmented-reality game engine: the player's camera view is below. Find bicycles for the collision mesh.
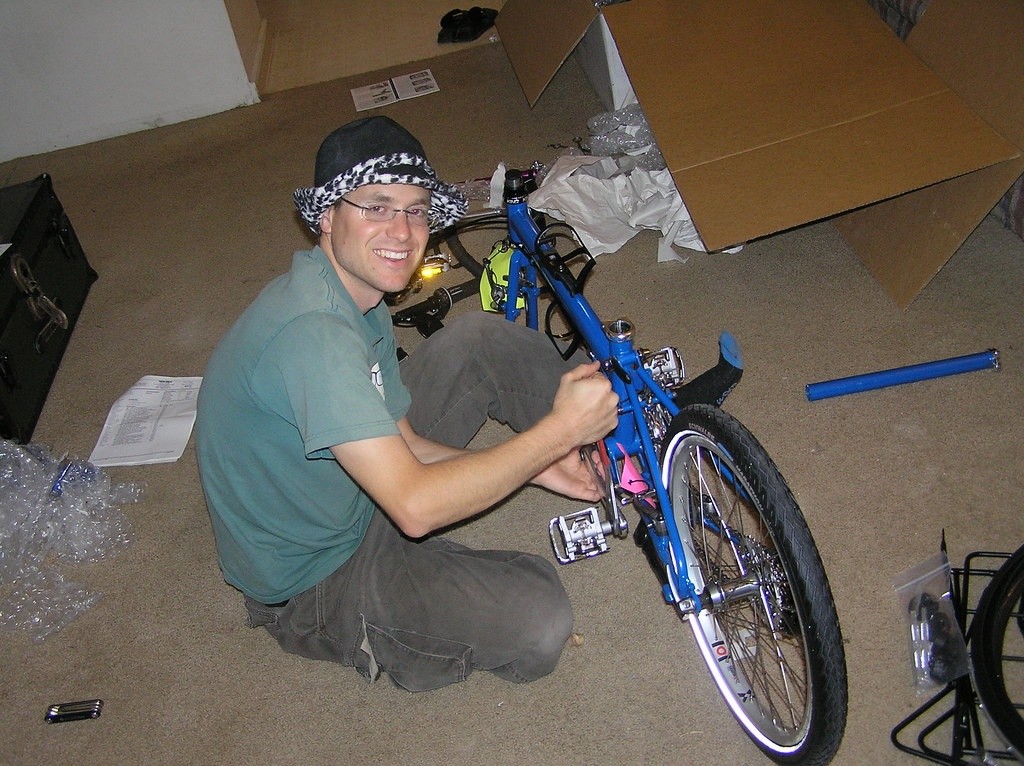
[379,167,851,766]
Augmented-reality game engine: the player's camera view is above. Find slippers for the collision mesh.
[453,6,498,48]
[438,8,468,44]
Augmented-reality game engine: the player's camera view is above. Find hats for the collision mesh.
[294,116,470,237]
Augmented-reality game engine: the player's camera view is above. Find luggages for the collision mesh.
[0,174,98,450]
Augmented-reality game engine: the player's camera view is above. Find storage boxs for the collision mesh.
[0,174,99,443]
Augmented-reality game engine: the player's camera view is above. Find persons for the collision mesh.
[194,115,741,693]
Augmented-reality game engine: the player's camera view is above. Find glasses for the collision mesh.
[340,196,441,227]
